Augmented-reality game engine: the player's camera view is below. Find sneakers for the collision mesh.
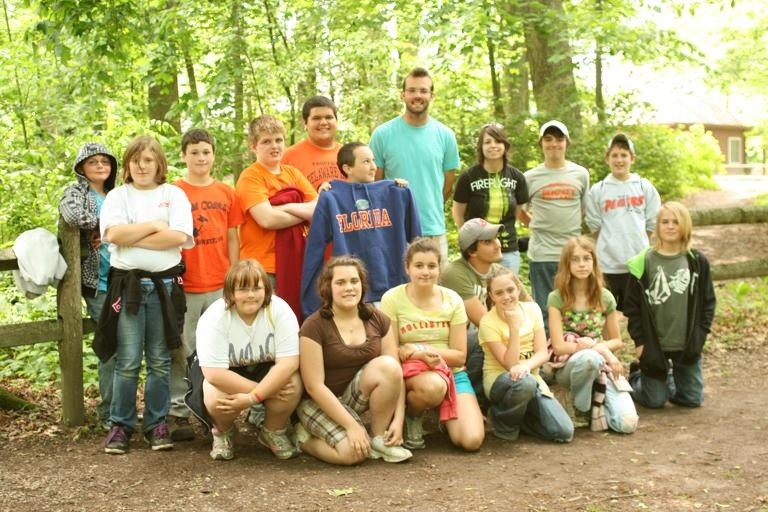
[287,423,309,453]
[167,415,194,440]
[248,410,265,427]
[370,430,412,463]
[565,395,590,427]
[144,424,174,450]
[211,424,237,460]
[259,424,293,460]
[404,418,425,449]
[104,426,128,454]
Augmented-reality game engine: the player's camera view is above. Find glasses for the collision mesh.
[236,287,265,292]
[405,89,432,95]
[87,159,110,165]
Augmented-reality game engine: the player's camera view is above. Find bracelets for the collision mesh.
[252,388,263,403]
[248,392,257,405]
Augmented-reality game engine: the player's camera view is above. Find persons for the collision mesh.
[478,268,575,444]
[288,253,414,465]
[548,236,640,434]
[196,257,304,461]
[584,134,662,306]
[281,96,349,196]
[369,68,459,275]
[57,143,117,431]
[166,128,245,440]
[318,141,411,310]
[622,201,717,408]
[234,113,332,294]
[516,119,590,340]
[99,135,196,454]
[378,235,485,452]
[452,121,529,277]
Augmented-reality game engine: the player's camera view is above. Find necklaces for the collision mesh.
[235,314,259,344]
[330,308,359,334]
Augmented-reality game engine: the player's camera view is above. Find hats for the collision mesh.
[458,218,504,252]
[540,120,569,140]
[608,134,635,153]
